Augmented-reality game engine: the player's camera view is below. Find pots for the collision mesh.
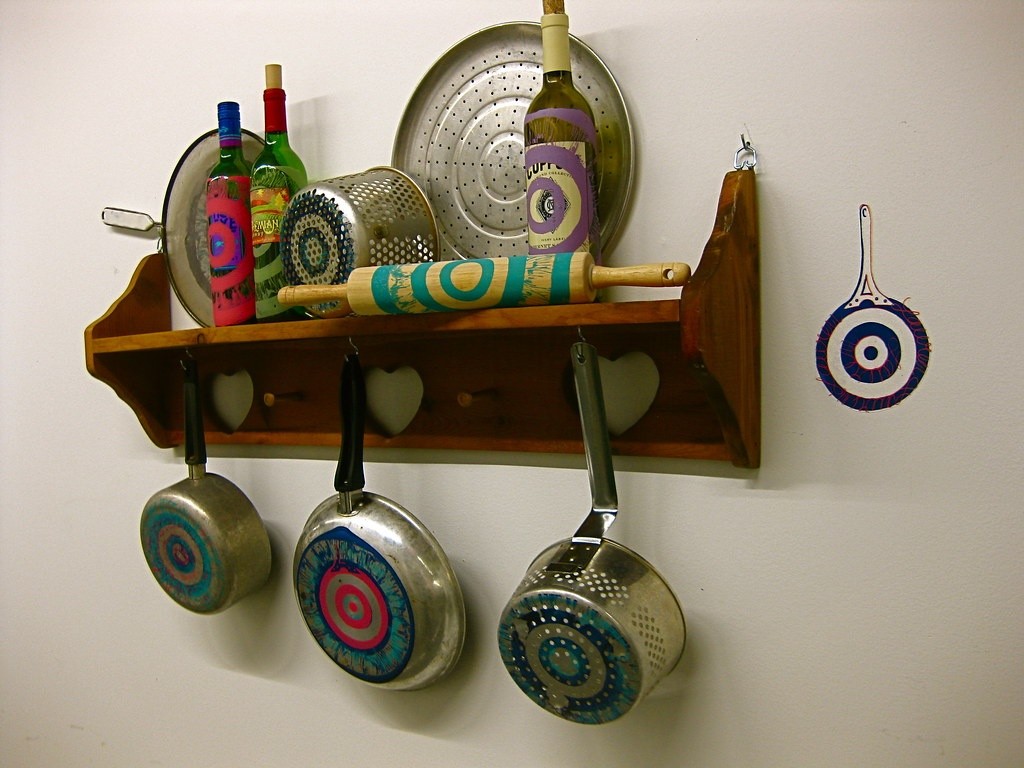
[294,351,467,692]
[139,361,270,615]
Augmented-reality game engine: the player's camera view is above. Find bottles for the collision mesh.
[205,101,254,327]
[250,88,308,322]
[524,14,602,267]
